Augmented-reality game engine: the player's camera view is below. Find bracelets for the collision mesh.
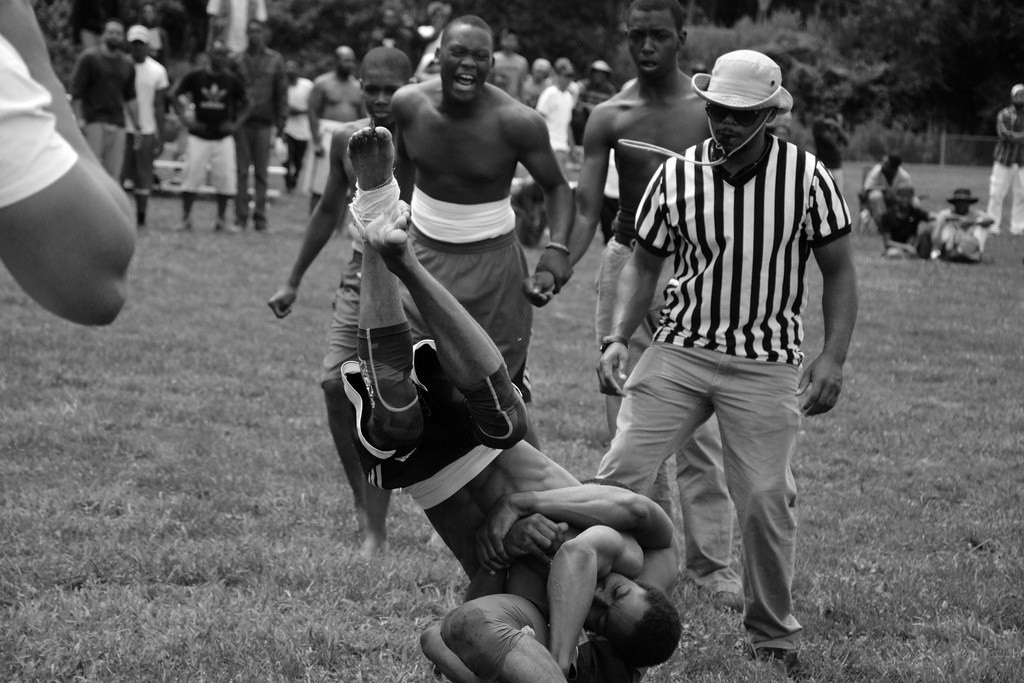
[545,240,571,255]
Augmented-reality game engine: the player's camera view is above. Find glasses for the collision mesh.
[705,103,767,126]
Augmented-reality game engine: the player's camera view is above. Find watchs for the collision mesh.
[599,334,630,353]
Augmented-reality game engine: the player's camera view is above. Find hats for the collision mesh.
[691,48,794,115]
[1010,83,1024,96]
[588,59,612,76]
[126,24,152,45]
[947,188,979,204]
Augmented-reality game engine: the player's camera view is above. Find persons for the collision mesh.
[171,40,253,232]
[2,0,137,327]
[265,47,416,557]
[70,18,142,185]
[520,1,745,614]
[125,23,171,226]
[142,4,172,116]
[71,21,102,77]
[986,84,1024,237]
[279,0,616,219]
[340,124,683,668]
[592,48,860,682]
[228,18,288,232]
[858,153,915,236]
[811,96,853,193]
[877,185,937,260]
[349,13,575,548]
[205,0,269,65]
[928,188,996,264]
[418,479,681,682]
